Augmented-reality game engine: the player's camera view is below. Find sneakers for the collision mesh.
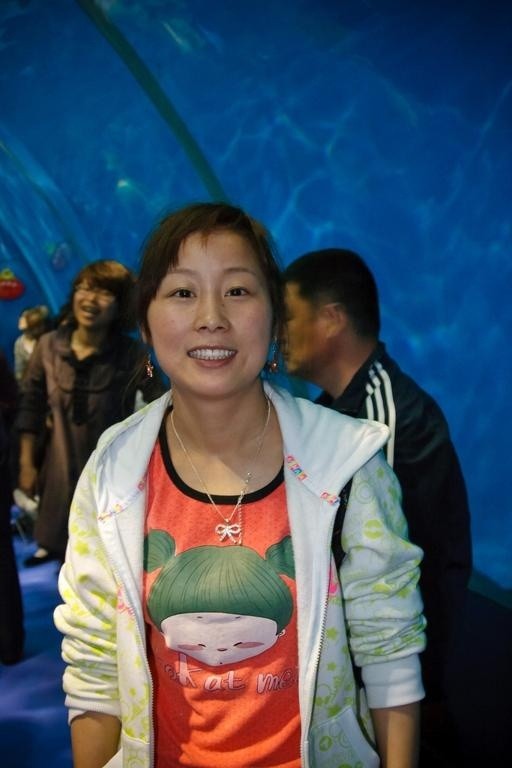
[23,545,58,571]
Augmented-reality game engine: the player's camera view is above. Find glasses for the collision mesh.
[72,283,119,303]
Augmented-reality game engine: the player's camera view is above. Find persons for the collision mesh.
[10,307,65,566]
[52,202,429,768]
[15,260,166,582]
[278,247,473,733]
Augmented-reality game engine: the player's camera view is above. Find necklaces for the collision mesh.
[167,397,274,543]
